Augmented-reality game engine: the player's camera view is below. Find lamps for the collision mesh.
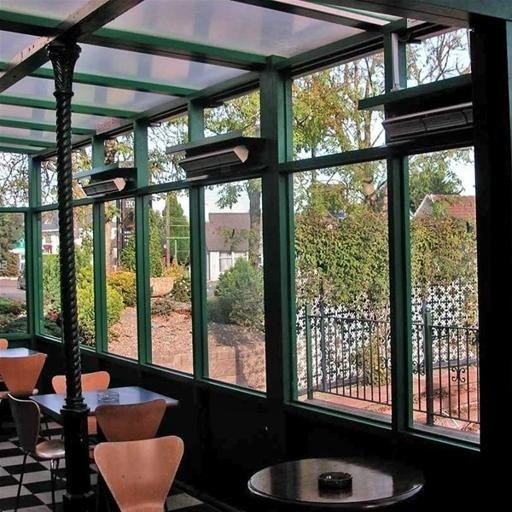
[82,175,128,198]
[380,100,472,147]
[177,143,250,179]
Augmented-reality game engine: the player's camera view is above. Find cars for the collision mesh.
[17,264,27,290]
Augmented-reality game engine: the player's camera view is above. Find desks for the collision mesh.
[245,453,427,511]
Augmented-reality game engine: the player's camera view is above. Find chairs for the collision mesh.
[0,336,187,511]
